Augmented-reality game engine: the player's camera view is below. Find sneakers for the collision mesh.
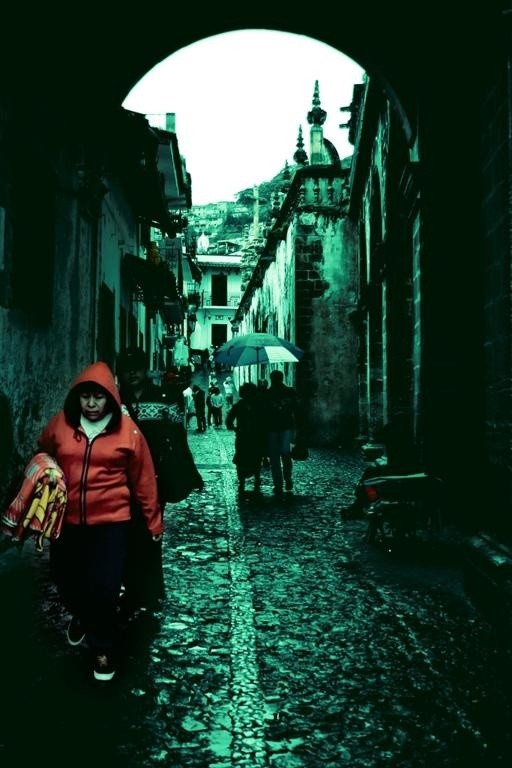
[196,422,221,432]
[67,613,85,646]
[272,480,292,493]
[93,654,116,681]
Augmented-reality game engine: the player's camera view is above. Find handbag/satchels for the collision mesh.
[292,446,309,460]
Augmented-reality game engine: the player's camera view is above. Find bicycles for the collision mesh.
[226,397,231,411]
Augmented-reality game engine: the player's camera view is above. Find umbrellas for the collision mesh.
[210,332,307,378]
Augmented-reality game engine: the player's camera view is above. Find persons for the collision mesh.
[226,382,265,502]
[165,341,235,433]
[30,362,165,683]
[257,378,270,469]
[267,370,304,494]
[115,345,206,623]
